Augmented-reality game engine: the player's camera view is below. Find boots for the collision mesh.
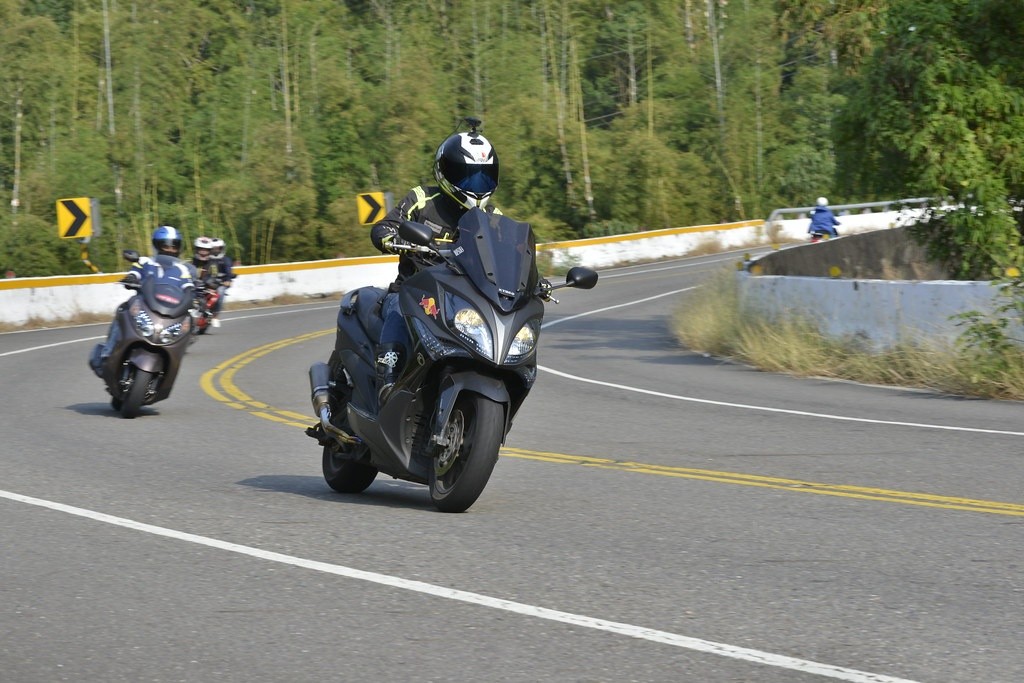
[375,341,407,410]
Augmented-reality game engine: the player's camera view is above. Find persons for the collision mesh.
[807,197,839,238]
[184,237,231,318]
[371,131,552,411]
[100,226,217,363]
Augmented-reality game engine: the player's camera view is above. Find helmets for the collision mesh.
[816,197,829,207]
[434,131,499,211]
[209,238,226,261]
[194,236,213,262]
[152,225,183,250]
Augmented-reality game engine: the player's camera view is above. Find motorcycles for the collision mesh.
[188,261,237,337]
[305,206,599,511]
[809,210,832,244]
[90,250,217,420]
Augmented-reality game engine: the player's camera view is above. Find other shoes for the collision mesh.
[100,348,110,360]
[211,318,221,328]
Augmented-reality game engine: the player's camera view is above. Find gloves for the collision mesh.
[119,273,137,290]
[536,278,553,302]
[197,293,207,313]
[388,233,418,258]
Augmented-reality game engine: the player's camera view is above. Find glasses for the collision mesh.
[160,241,180,250]
